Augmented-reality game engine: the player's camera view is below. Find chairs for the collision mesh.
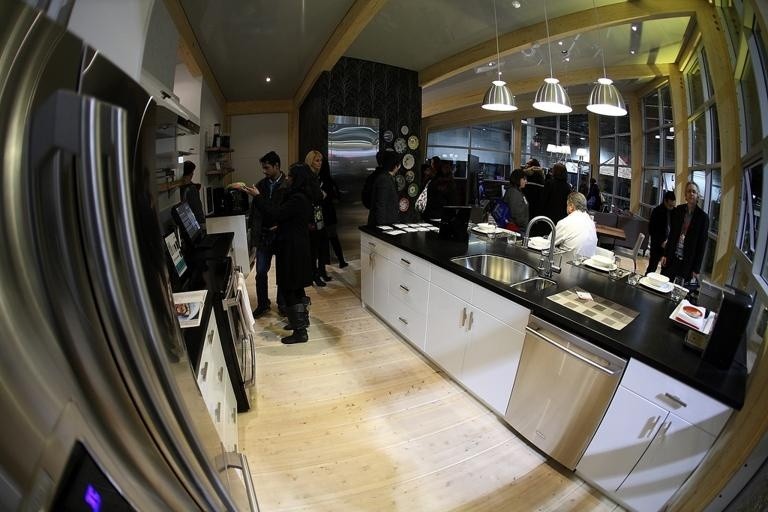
[613,232,644,272]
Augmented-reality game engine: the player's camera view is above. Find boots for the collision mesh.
[282,305,308,344]
[284,296,311,329]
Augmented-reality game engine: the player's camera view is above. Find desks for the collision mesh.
[594,224,625,250]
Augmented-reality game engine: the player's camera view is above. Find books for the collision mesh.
[171,289,209,329]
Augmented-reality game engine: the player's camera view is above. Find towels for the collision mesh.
[233,273,255,340]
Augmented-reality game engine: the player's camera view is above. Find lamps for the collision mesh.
[481,0,517,113]
[532,0,574,114]
[585,0,628,118]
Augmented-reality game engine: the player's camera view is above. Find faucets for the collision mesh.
[522,215,558,262]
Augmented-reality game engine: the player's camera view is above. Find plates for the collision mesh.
[176,302,198,320]
[468,220,623,281]
[638,276,674,293]
[383,125,421,213]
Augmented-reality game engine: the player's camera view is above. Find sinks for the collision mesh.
[452,253,539,286]
[511,277,558,298]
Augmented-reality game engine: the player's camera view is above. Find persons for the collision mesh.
[661,181,709,283]
[505,157,603,254]
[421,155,453,218]
[243,163,315,343]
[366,152,401,224]
[181,160,202,221]
[644,191,675,274]
[360,151,383,208]
[307,151,331,286]
[321,160,347,269]
[247,152,289,318]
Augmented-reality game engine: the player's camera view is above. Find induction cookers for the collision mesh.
[169,255,234,298]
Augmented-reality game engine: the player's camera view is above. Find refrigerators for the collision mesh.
[0,0,265,512]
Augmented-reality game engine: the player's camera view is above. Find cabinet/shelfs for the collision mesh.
[195,307,245,486]
[573,357,732,512]
[423,261,532,416]
[358,229,430,356]
[204,145,235,176]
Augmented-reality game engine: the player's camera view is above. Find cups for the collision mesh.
[626,268,637,286]
[670,274,688,301]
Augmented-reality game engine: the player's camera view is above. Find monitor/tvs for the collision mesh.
[171,199,203,251]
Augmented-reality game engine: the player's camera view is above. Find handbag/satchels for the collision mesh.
[414,187,427,213]
[492,200,511,227]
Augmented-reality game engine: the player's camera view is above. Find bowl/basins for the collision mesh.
[645,272,669,286]
[682,305,703,319]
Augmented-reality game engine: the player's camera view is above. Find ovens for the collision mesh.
[213,265,256,388]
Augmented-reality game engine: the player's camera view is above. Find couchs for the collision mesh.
[558,209,656,256]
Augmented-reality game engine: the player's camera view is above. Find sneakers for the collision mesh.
[252,302,271,318]
[278,305,286,316]
[339,263,348,269]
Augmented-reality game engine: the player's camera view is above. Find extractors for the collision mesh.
[140,68,198,140]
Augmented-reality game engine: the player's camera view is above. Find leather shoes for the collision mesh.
[318,269,333,282]
[313,273,327,287]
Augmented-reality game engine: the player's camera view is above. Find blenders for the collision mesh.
[211,123,221,147]
